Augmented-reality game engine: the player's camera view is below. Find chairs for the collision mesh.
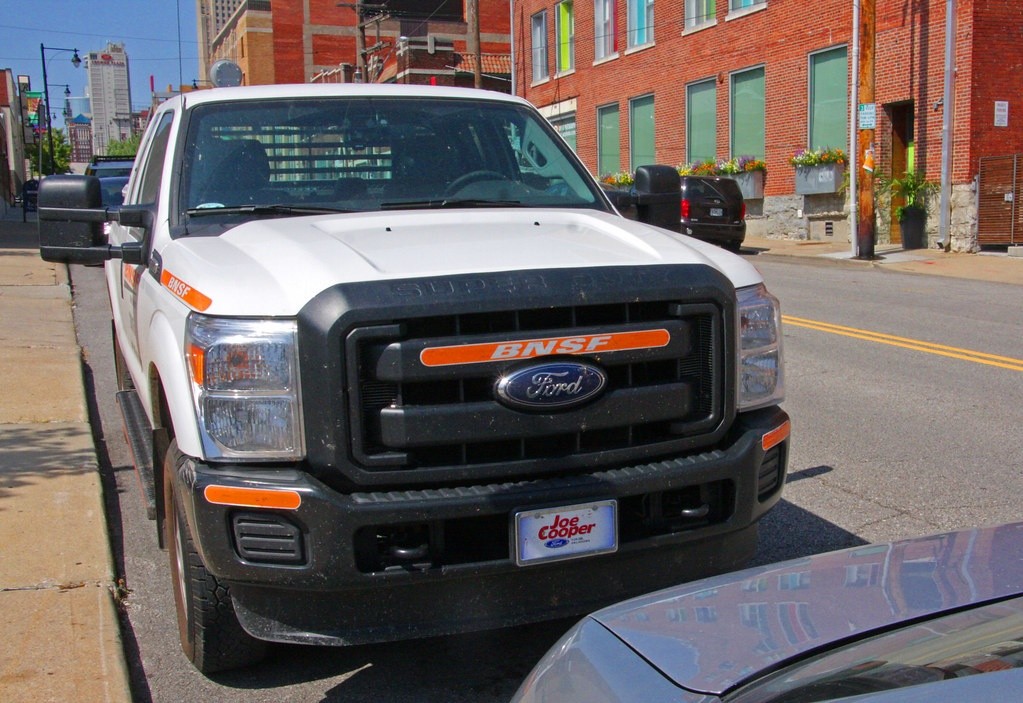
[194,138,292,208]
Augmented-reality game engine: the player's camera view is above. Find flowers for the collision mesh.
[597,156,767,188]
[788,147,849,170]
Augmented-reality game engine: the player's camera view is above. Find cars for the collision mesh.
[94,178,133,211]
[621,174,747,251]
[504,511,1022,703]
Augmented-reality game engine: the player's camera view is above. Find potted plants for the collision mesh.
[875,167,942,250]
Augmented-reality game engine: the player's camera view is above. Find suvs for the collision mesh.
[36,82,790,685]
[82,153,137,180]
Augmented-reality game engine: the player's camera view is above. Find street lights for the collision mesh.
[39,43,82,176]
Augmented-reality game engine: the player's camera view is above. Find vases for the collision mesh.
[718,169,765,200]
[794,161,845,195]
